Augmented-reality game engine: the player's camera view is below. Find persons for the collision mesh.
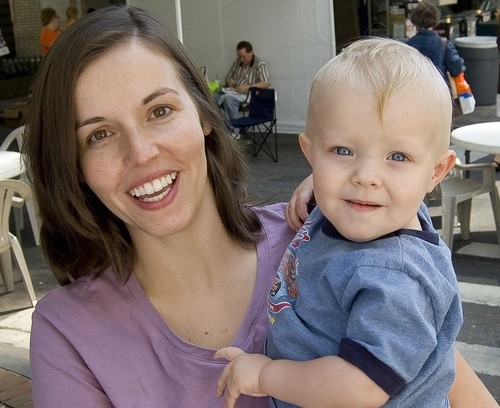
[59,6,96,33]
[214,40,271,140]
[404,2,466,90]
[39,7,61,55]
[21,4,499,408]
[212,36,464,408]
[492,154,500,173]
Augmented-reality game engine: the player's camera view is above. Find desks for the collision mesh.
[0,151,29,236]
[451,121,500,179]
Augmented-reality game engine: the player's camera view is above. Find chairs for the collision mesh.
[0,124,44,244]
[226,87,278,163]
[0,179,37,308]
[423,162,500,252]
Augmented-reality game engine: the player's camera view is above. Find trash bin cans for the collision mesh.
[454,36,498,106]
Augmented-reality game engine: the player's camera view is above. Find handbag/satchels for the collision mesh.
[439,37,477,116]
